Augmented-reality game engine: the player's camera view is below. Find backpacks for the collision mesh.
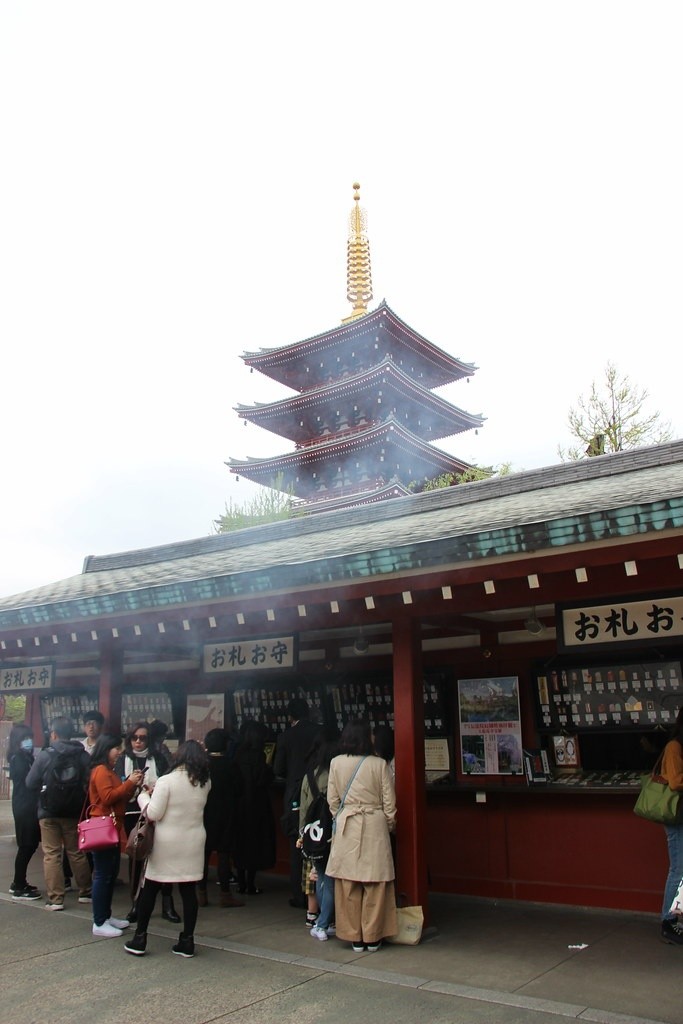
[300,770,333,860]
[38,745,87,816]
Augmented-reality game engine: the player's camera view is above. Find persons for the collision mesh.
[652,706,683,948]
[7,724,42,902]
[123,739,211,958]
[25,717,91,911]
[63,710,105,891]
[296,719,398,952]
[197,699,322,909]
[88,720,181,938]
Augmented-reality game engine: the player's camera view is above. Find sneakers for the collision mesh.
[45,902,64,911]
[78,894,92,902]
[109,916,129,929]
[660,916,683,947]
[9,882,37,894]
[307,911,381,951]
[12,886,42,901]
[92,919,122,937]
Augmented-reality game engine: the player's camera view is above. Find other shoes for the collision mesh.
[65,878,72,891]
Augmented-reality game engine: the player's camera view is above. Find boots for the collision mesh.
[238,870,246,893]
[127,896,138,922]
[172,932,194,957]
[196,889,207,906]
[220,892,245,907]
[124,931,147,955]
[162,895,181,922]
[248,870,262,894]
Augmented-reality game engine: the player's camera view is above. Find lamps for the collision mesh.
[524,603,546,639]
[353,624,370,656]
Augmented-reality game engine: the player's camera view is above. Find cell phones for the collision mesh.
[139,767,149,774]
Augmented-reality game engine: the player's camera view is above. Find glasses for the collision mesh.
[131,734,147,742]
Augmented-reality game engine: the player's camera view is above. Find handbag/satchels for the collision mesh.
[125,803,154,861]
[77,804,120,852]
[633,751,683,825]
[384,904,424,945]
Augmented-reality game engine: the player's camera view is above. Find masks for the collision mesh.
[20,739,33,750]
